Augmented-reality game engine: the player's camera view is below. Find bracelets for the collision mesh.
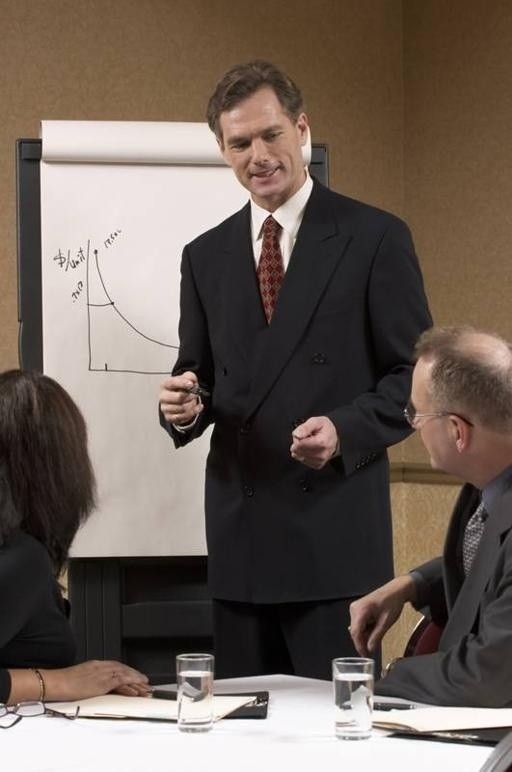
[29,665,47,703]
[382,656,404,678]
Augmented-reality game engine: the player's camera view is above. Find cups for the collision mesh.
[329,656,377,740]
[175,651,217,733]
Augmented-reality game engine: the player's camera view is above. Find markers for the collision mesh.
[171,385,211,397]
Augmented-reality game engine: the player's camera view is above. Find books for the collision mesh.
[371,708,511,733]
[47,692,257,723]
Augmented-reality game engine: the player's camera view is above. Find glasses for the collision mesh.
[403,400,476,430]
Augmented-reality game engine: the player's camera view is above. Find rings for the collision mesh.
[347,625,352,632]
[300,456,305,463]
[112,671,118,678]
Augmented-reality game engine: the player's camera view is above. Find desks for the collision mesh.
[1,672,512,772]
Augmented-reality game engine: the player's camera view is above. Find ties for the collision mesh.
[462,501,486,579]
[256,216,282,326]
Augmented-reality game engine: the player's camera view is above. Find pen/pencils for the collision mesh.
[371,701,414,711]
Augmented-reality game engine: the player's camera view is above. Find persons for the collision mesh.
[347,321,512,711]
[156,58,432,682]
[0,368,153,715]
